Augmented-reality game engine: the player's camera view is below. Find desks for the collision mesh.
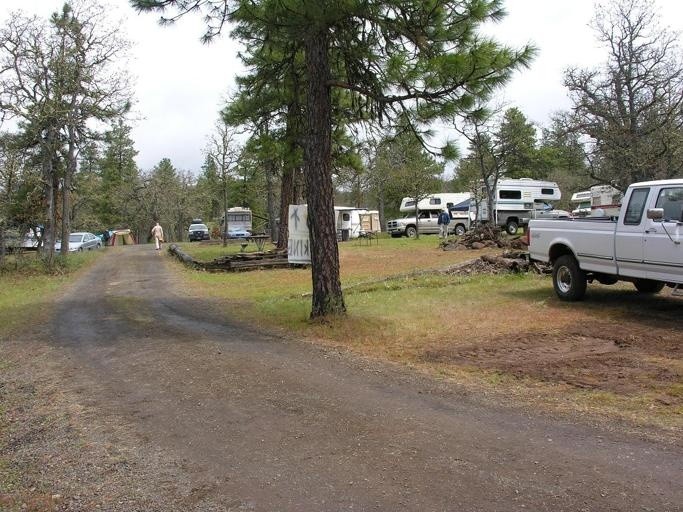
[358,230,378,247]
[241,236,269,251]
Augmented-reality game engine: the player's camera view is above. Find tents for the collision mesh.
[108,229,136,246]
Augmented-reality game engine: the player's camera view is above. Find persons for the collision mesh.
[438,208,451,239]
[151,222,163,250]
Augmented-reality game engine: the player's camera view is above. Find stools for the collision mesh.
[241,244,248,251]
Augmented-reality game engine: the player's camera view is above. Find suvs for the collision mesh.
[187,217,210,242]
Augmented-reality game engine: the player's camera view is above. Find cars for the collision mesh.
[55,231,103,252]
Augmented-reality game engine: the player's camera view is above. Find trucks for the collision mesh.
[218,207,252,240]
[386,191,475,236]
[570,190,592,218]
[590,182,624,219]
[474,176,574,230]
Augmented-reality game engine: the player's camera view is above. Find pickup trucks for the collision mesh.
[525,176,683,301]
